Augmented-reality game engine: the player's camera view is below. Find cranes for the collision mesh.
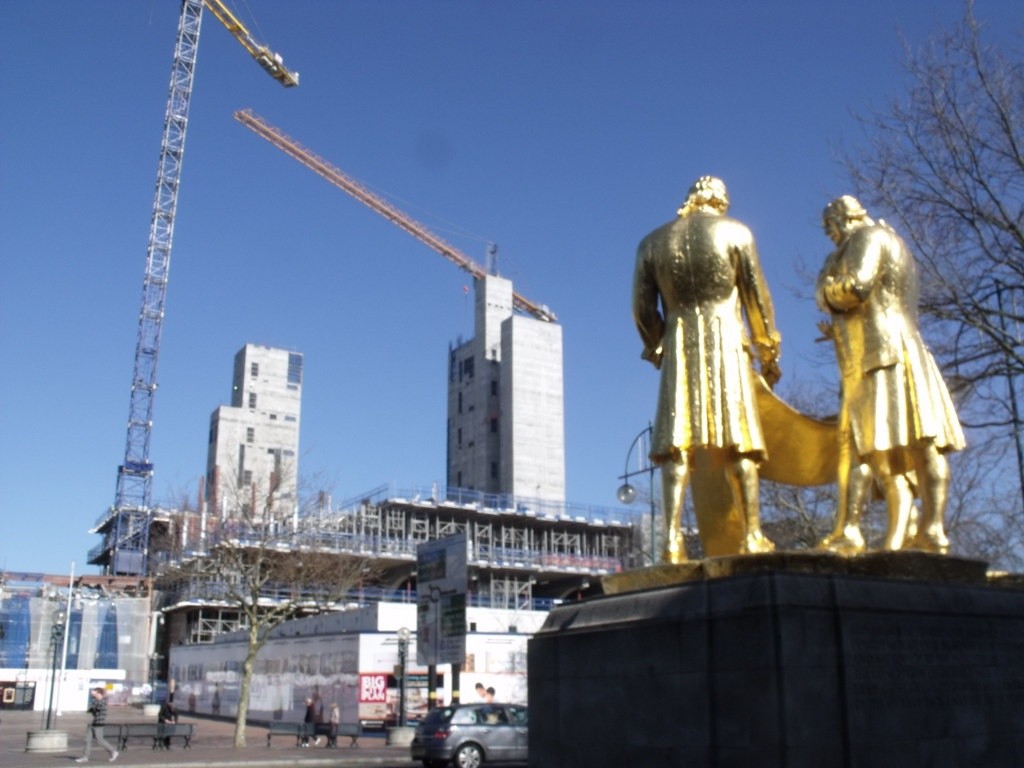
[108,0,302,579]
[231,104,564,332]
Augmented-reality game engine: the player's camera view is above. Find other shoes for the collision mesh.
[314,737,322,747]
[75,756,89,763]
[301,743,310,748]
[108,751,119,762]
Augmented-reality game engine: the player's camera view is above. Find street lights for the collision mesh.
[42,604,70,733]
[616,416,667,566]
[943,276,1023,536]
[397,628,410,729]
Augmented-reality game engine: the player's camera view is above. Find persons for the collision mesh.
[212,683,221,717]
[325,703,340,747]
[302,698,321,748]
[486,686,496,703]
[158,703,178,749]
[807,195,967,559]
[475,683,485,704]
[632,176,783,564]
[74,688,120,763]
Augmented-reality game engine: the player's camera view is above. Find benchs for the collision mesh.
[92,723,197,752]
[267,723,365,750]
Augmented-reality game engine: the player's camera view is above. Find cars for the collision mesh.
[407,703,529,768]
